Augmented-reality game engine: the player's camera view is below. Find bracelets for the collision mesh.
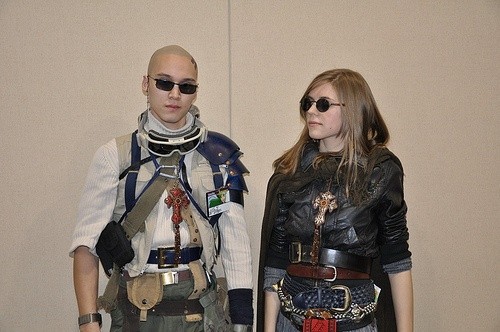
[78,313,102,327]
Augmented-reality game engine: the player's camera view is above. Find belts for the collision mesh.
[130,268,192,287]
[288,241,372,274]
[145,246,204,270]
[281,273,376,312]
[280,309,374,332]
[285,263,371,283]
[119,298,204,319]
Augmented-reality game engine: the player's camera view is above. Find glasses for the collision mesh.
[299,97,346,113]
[148,74,199,95]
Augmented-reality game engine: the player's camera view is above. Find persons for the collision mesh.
[67,46,254,332]
[257,68,416,332]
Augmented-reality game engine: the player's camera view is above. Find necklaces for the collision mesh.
[310,191,338,264]
[149,152,189,263]
